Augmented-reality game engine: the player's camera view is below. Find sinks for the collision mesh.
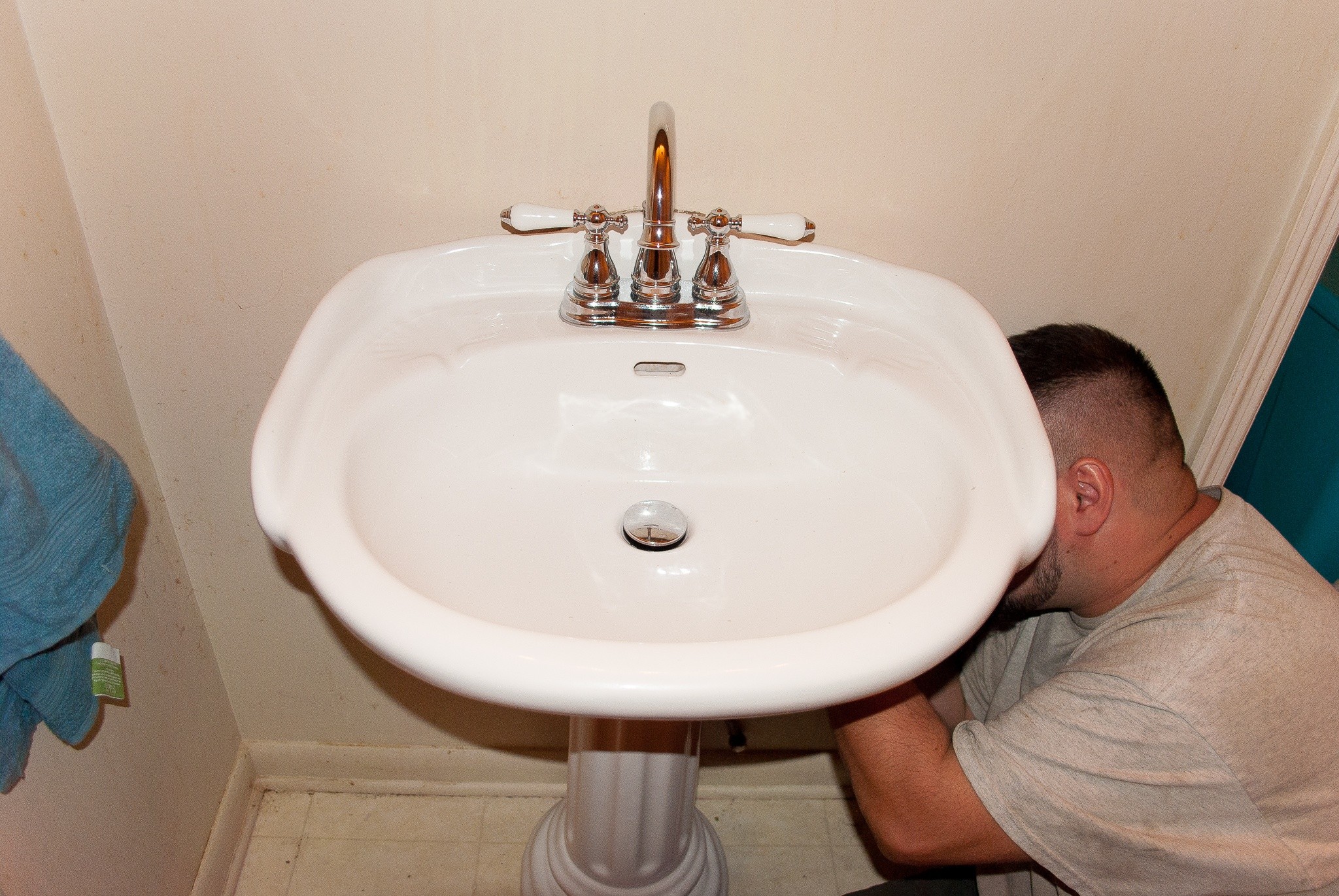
[252,222,1057,724]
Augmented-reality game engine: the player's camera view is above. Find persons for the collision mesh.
[827,323,1338,896]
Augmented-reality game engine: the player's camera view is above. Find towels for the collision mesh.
[0,340,141,801]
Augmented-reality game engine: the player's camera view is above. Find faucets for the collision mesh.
[501,101,815,331]
